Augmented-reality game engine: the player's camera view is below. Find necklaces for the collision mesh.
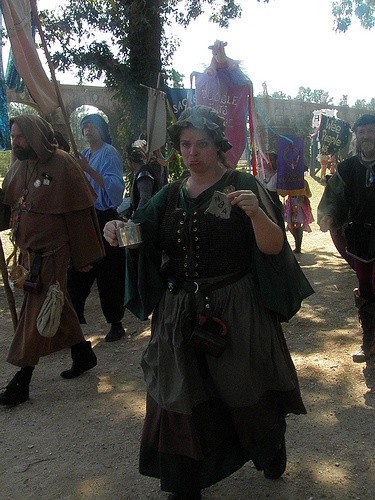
[10,157,38,238]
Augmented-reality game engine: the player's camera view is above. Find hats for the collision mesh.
[168,105,232,154]
[131,139,153,163]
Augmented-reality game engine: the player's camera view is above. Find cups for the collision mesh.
[189,314,230,358]
[23,274,44,295]
[117,223,143,248]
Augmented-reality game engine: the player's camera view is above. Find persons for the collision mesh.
[68,112,125,343]
[264,152,312,253]
[125,144,158,207]
[0,114,104,406]
[316,114,375,361]
[102,104,315,500]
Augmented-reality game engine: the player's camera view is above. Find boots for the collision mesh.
[59,340,98,380]
[0,370,31,406]
[292,240,302,253]
[352,288,375,363]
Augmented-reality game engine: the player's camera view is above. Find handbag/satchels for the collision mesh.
[37,281,64,337]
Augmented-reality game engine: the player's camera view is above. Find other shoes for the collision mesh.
[104,322,126,342]
[264,434,287,479]
